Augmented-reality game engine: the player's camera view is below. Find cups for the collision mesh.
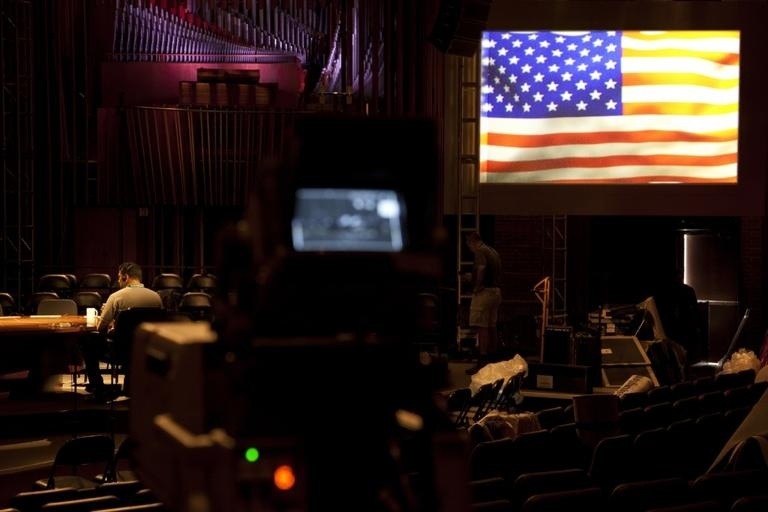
[86,308,99,318]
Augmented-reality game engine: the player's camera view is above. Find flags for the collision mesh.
[479,28,742,185]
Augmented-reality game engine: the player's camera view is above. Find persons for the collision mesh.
[464,232,503,374]
[83,263,163,393]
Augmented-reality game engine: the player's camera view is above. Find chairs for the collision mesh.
[470,368,768,512]
[0,274,215,512]
